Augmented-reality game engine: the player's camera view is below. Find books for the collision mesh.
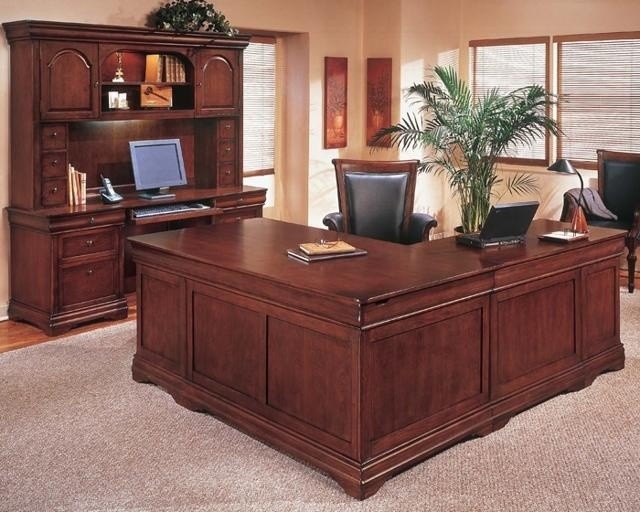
[67,162,87,207]
[142,52,188,83]
[298,240,356,255]
[287,246,370,263]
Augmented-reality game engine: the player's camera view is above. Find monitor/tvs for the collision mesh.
[128,139,188,200]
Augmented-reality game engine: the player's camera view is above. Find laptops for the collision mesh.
[456,201,540,248]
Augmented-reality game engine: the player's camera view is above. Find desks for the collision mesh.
[125,217,630,502]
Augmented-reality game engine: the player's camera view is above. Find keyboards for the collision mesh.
[128,202,210,218]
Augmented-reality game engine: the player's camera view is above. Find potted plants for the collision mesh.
[366,62,571,236]
[144,0,240,40]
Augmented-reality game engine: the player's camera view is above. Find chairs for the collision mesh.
[322,158,438,245]
[563,148,640,294]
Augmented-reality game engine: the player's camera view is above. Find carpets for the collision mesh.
[1,285,640,510]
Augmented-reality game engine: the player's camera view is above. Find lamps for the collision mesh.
[546,157,590,235]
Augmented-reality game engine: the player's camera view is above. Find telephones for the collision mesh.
[100,173,124,204]
